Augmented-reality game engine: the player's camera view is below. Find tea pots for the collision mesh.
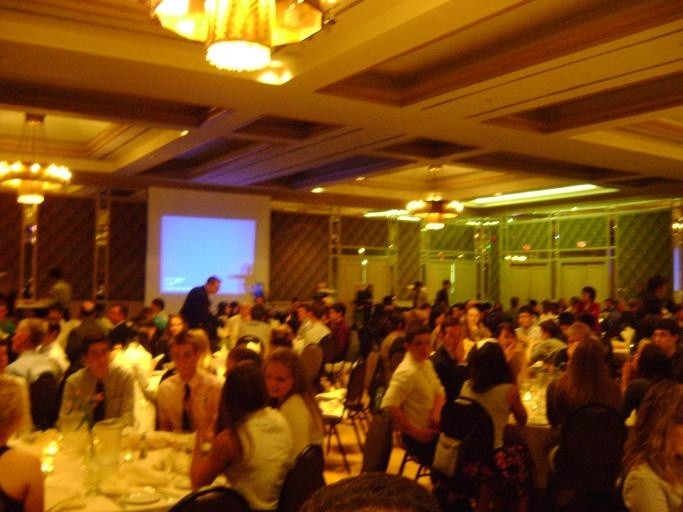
[94,417,126,497]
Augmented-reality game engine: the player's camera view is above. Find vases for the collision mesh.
[83,434,99,494]
[554,402,626,495]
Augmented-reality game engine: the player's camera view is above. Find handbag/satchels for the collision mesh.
[430,431,463,478]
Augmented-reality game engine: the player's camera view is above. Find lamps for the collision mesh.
[147,0,330,86]
[1,113,75,204]
[405,192,464,231]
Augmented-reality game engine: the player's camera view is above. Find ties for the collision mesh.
[89,380,106,428]
[181,383,191,434]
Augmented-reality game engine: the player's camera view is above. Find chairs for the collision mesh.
[441,397,494,474]
[386,351,431,480]
[279,445,325,511]
[320,333,337,367]
[302,343,322,381]
[345,330,361,361]
[172,488,251,511]
[366,345,383,425]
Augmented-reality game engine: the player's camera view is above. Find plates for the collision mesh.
[118,485,162,506]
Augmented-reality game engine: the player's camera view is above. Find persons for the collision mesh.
[463,286,683,330]
[492,321,524,375]
[178,276,226,329]
[429,317,468,403]
[380,324,446,466]
[217,281,349,346]
[12,309,33,354]
[4,317,61,384]
[554,323,595,367]
[49,304,65,319]
[190,362,293,512]
[262,347,324,469]
[457,342,534,490]
[1,271,15,321]
[161,316,185,343]
[225,346,262,380]
[132,307,157,344]
[54,335,135,432]
[65,300,107,361]
[36,320,69,371]
[300,473,437,512]
[109,301,135,349]
[350,279,462,326]
[152,297,170,329]
[622,345,673,419]
[155,333,216,433]
[0,374,44,512]
[49,267,72,321]
[653,319,682,380]
[545,337,622,472]
[621,379,683,512]
[528,319,566,362]
